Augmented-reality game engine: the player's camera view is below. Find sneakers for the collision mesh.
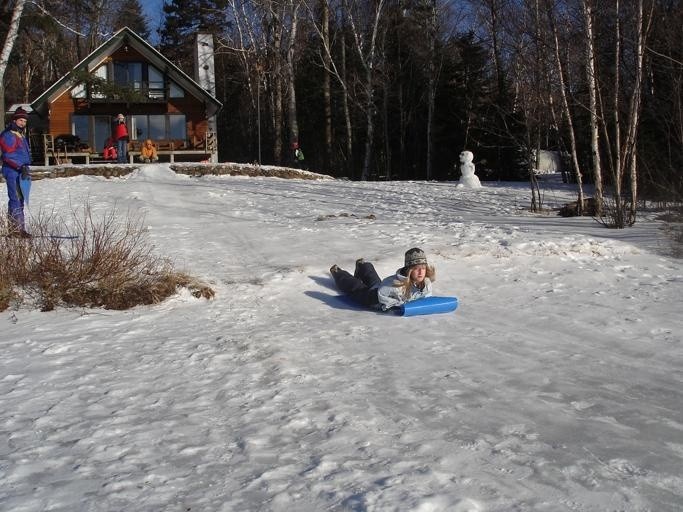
[330,264,342,279]
[104,157,128,164]
[8,230,33,239]
[354,258,365,277]
[144,158,155,163]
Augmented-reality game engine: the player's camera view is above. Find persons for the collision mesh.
[298,149,309,170]
[103,137,117,160]
[111,114,130,164]
[138,140,158,163]
[0,106,33,237]
[328,246,437,314]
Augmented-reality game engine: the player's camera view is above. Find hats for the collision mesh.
[12,106,29,120]
[117,114,124,118]
[405,248,427,267]
